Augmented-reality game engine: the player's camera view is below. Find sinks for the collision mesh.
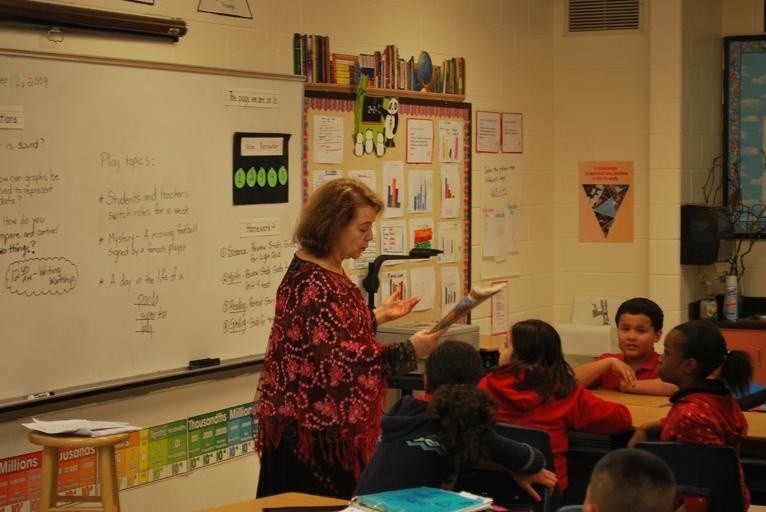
[744,313,766,324]
[550,317,623,359]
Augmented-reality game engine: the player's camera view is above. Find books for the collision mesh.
[427,283,507,335]
[294,33,465,97]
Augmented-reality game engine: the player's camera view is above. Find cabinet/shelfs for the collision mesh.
[717,327,766,391]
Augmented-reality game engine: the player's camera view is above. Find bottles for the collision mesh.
[701,280,718,320]
[724,274,739,323]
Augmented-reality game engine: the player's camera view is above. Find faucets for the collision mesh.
[591,298,611,325]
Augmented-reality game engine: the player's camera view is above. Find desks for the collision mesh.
[587,387,766,441]
[195,491,352,512]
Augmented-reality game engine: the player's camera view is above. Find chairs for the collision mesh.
[491,421,566,512]
[637,440,745,512]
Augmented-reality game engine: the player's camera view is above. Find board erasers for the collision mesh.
[189,358,220,370]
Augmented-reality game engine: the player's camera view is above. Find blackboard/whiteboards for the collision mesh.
[0,47,306,410]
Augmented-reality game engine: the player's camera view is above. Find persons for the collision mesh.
[349,340,559,504]
[251,179,455,511]
[476,319,632,499]
[569,298,678,397]
[583,450,677,512]
[627,320,751,512]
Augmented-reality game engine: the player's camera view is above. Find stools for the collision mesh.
[28,431,131,512]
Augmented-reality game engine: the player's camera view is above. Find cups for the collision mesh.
[478,348,500,369]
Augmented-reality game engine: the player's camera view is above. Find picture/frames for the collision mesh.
[197,0,252,18]
[128,0,155,5]
